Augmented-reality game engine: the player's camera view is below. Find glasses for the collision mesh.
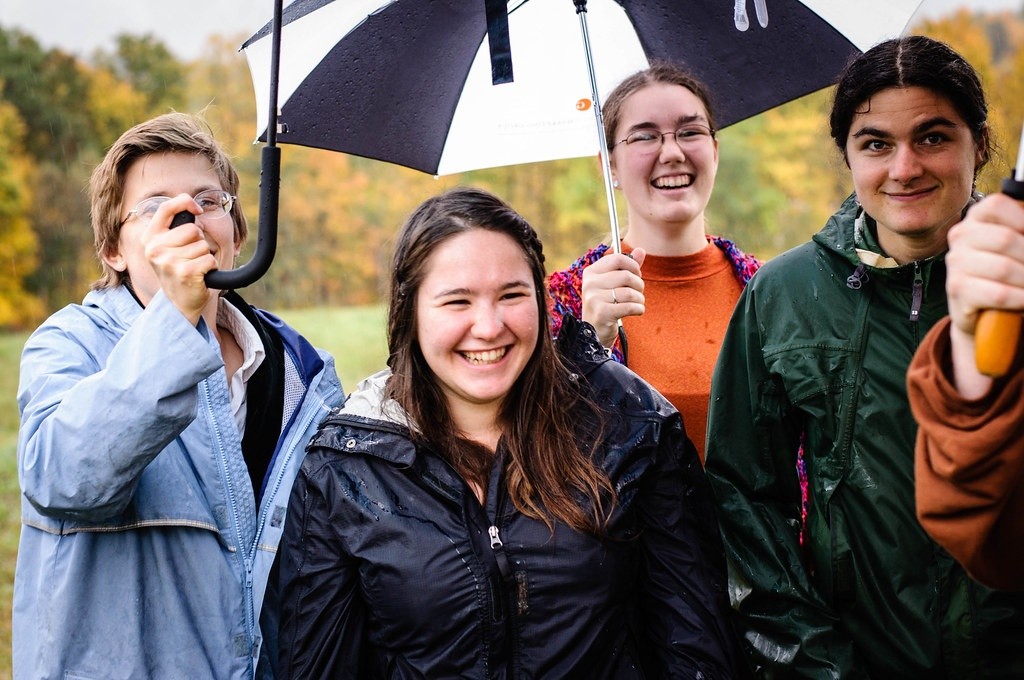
[113,188,238,231]
[614,123,717,155]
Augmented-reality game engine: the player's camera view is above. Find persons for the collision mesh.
[10,36,1024,679]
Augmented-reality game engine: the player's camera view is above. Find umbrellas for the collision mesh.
[169,1,922,290]
